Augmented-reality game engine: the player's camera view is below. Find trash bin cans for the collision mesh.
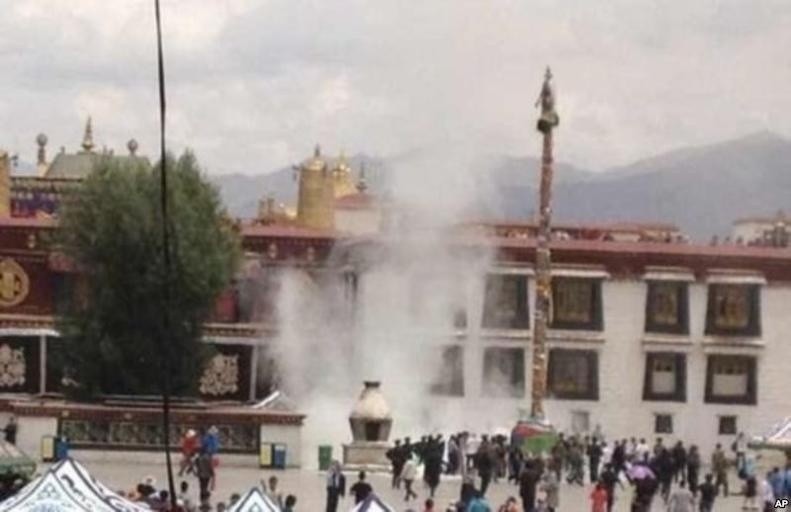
[41,436,69,462]
[318,446,332,470]
[260,442,287,469]
